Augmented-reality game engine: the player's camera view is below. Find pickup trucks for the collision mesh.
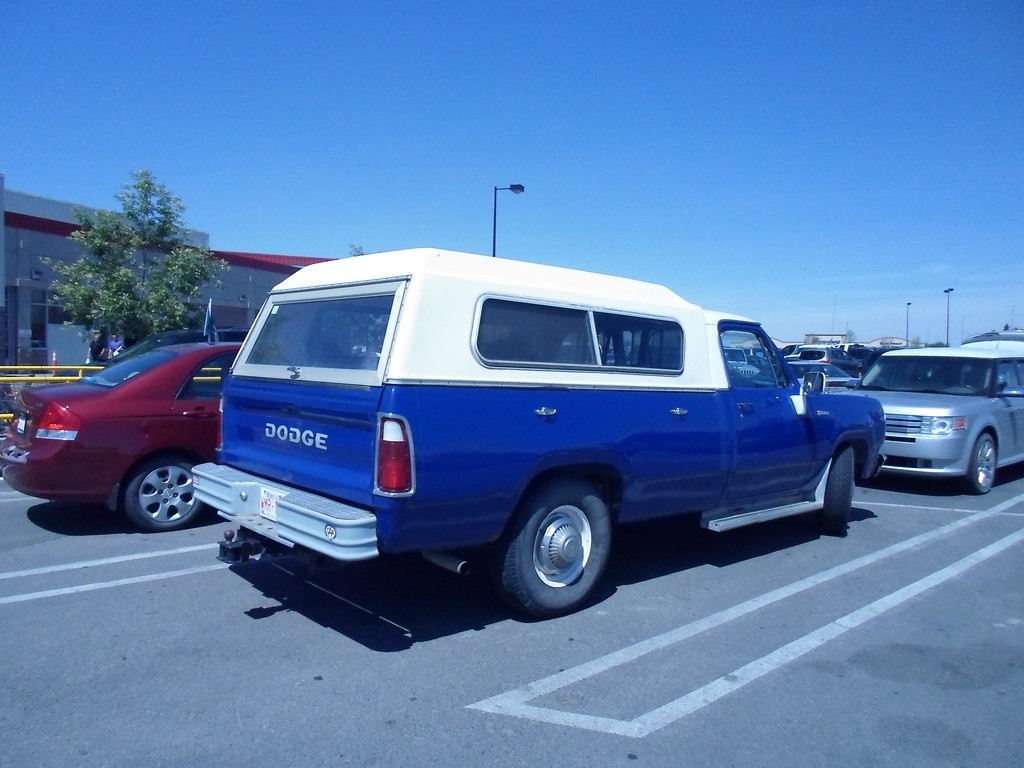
[189,246,890,622]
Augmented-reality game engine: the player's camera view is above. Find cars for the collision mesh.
[0,340,244,535]
[725,330,1023,394]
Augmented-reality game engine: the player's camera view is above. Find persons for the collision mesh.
[107,334,125,360]
[85,330,106,366]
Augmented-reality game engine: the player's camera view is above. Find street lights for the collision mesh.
[943,288,954,348]
[492,183,525,257]
[905,302,912,348]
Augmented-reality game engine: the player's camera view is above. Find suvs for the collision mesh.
[46,326,250,401]
[845,347,1024,495]
